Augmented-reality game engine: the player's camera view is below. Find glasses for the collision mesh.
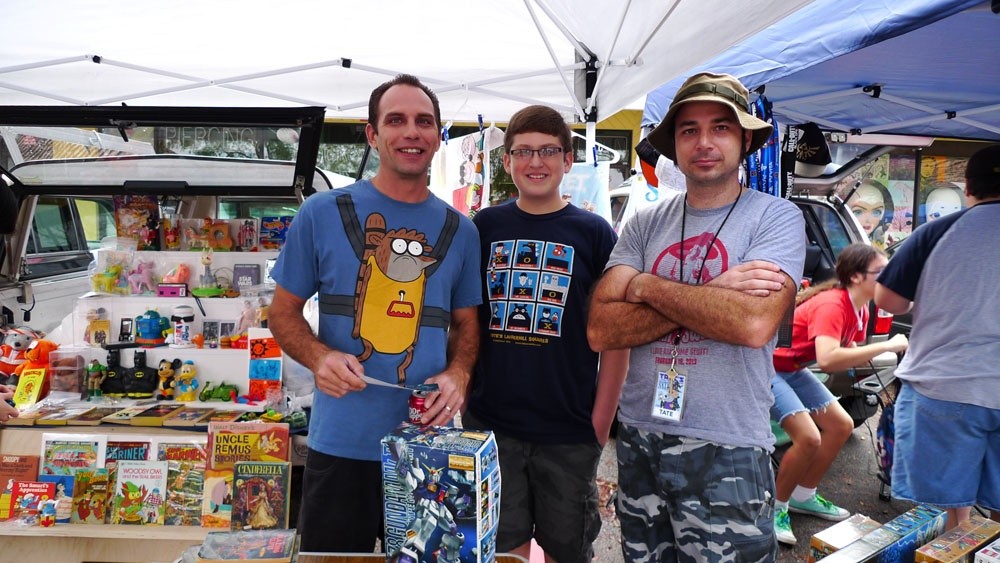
[509,148,566,161]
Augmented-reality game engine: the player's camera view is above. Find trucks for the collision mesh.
[0,156,356,394]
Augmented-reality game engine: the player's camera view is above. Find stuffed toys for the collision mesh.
[0,326,83,400]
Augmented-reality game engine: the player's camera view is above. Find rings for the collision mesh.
[446,406,451,412]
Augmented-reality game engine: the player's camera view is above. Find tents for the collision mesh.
[636,0,999,233]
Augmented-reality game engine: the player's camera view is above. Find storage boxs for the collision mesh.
[380,421,502,563]
[806,502,1000,563]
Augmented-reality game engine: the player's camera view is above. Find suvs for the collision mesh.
[609,175,896,378]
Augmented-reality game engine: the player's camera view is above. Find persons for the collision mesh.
[459,104,629,563]
[267,74,483,552]
[82,297,271,401]
[586,71,805,563]
[771,243,908,545]
[874,147,1000,534]
[0,385,19,421]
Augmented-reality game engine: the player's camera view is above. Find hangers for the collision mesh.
[571,130,620,164]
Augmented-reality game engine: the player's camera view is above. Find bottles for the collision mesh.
[170,305,195,349]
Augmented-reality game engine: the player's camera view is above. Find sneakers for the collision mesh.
[773,506,797,544]
[787,493,851,521]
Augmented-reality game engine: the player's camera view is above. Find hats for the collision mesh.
[647,72,774,160]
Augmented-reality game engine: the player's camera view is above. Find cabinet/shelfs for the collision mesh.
[0,251,319,563]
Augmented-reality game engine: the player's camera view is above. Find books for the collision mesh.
[0,422,301,563]
